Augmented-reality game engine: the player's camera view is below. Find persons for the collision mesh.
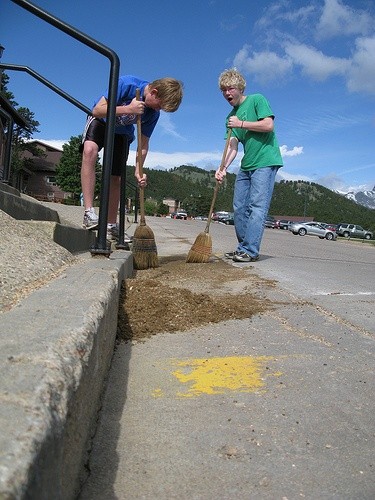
[78,75,184,242]
[216,67,284,263]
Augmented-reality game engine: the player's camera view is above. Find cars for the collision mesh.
[323,223,338,232]
[289,221,338,241]
[191,210,295,230]
[171,210,188,220]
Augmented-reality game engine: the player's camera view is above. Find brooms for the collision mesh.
[185,125,233,263]
[130,88,160,270]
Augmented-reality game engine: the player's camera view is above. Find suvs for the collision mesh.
[336,223,374,240]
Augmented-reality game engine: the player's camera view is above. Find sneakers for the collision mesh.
[232,253,256,262]
[81,208,98,230]
[106,225,133,243]
[224,250,260,259]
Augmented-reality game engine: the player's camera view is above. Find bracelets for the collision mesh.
[241,121,244,129]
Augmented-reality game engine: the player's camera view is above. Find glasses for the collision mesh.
[220,86,238,93]
